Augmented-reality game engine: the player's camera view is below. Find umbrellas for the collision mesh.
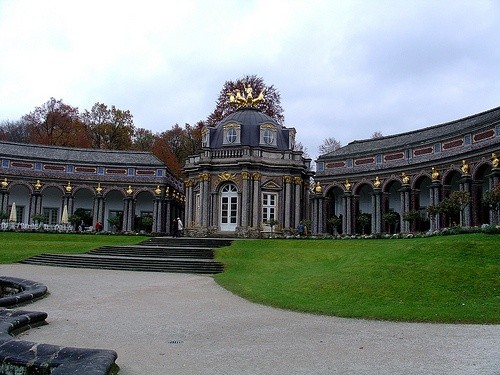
[62,204,68,230]
[9,202,16,222]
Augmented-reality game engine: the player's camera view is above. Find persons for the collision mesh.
[81,223,86,231]
[297,221,304,237]
[94,222,102,235]
[171,217,184,238]
